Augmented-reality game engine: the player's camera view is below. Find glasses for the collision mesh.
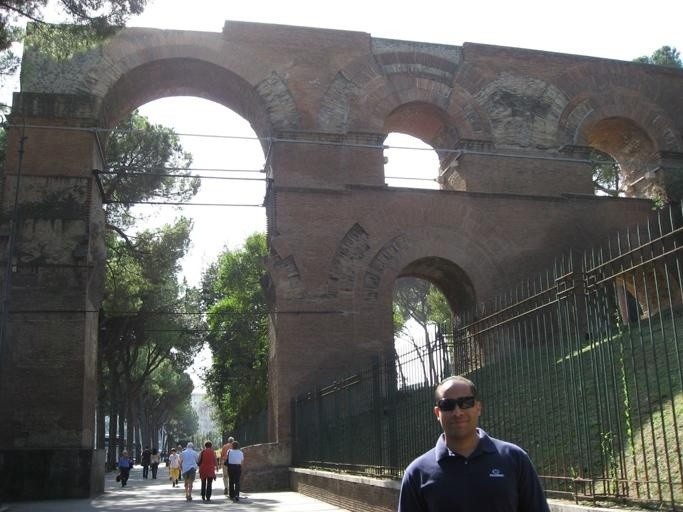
[435,396,474,412]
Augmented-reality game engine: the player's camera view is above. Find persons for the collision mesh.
[116,437,244,503]
[398,375,550,512]
